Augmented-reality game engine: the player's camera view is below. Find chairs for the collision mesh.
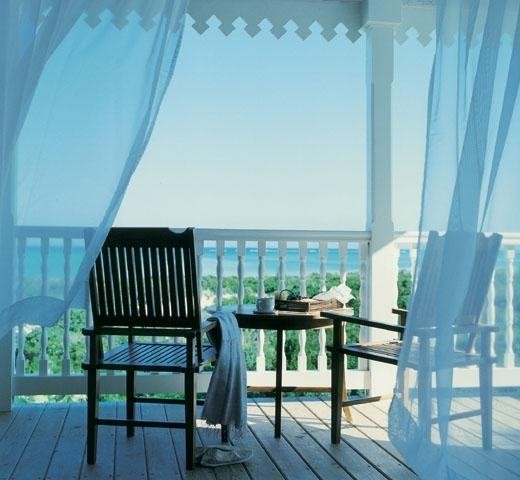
[79,225,237,470]
[320,228,505,455]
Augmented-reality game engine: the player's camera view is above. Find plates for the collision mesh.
[252,310,278,315]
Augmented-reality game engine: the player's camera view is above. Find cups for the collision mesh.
[256,298,276,312]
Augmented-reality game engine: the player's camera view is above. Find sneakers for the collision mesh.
[195,446,254,467]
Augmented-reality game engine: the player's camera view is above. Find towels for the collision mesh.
[288,284,356,312]
